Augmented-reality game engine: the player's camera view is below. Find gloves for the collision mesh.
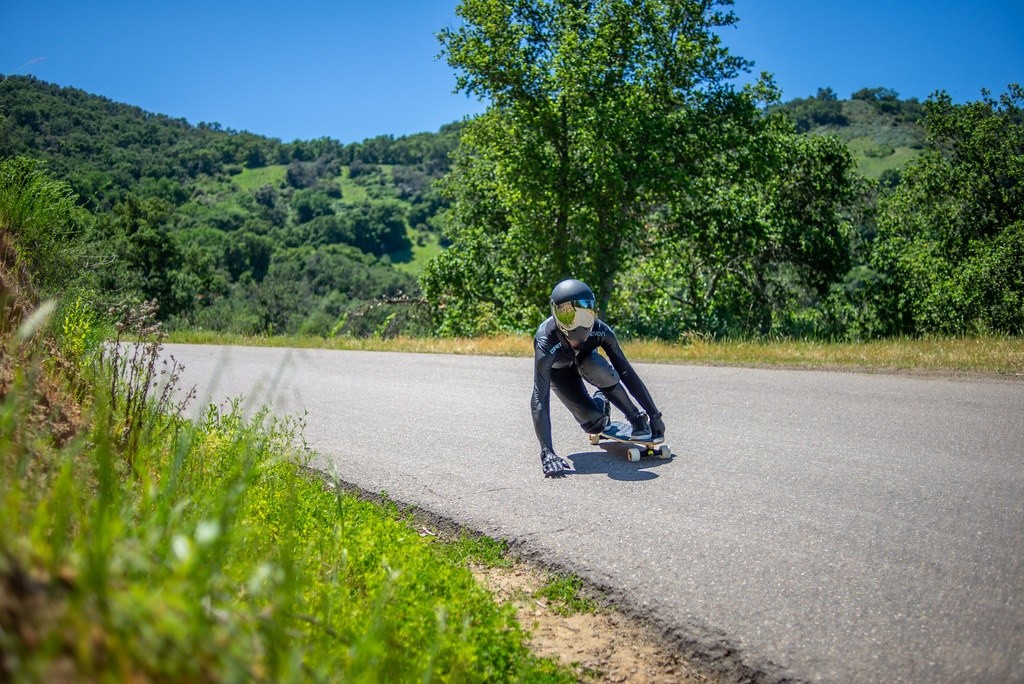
[649,412,665,438]
[540,449,570,475]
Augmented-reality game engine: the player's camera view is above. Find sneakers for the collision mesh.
[594,391,611,431]
[630,412,653,440]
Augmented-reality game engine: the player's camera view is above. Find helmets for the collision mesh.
[550,279,595,341]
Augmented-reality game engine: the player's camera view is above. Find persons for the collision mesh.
[530,279,665,474]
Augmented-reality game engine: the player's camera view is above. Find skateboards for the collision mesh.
[589,421,670,463]
